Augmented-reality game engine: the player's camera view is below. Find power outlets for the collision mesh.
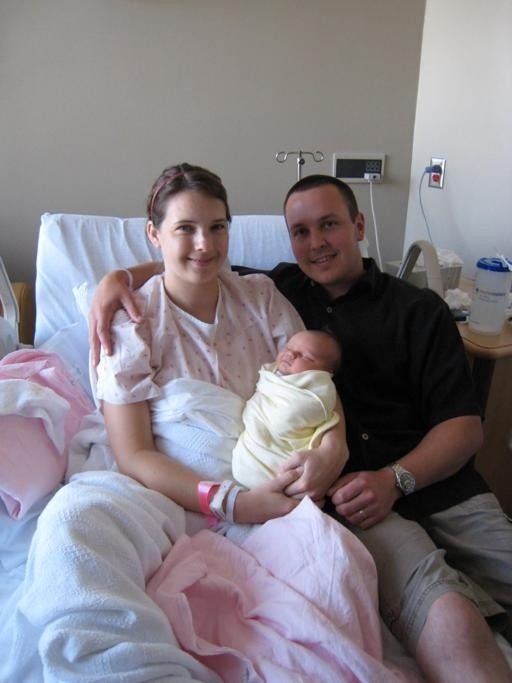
[428,158,446,188]
[333,153,386,184]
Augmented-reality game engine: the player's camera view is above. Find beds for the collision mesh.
[2,213,512,681]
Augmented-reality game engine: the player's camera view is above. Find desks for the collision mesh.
[447,275,512,436]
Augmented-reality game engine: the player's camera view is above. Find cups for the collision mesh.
[469,257,512,336]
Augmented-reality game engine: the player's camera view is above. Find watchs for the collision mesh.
[389,461,416,496]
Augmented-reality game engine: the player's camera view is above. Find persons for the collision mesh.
[231,324,342,490]
[30,162,379,683]
[87,175,512,683]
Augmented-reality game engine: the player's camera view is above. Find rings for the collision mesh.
[360,511,367,522]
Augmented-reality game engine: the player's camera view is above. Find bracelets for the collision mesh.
[225,485,242,524]
[116,266,134,292]
[211,479,233,520]
[197,478,218,528]
